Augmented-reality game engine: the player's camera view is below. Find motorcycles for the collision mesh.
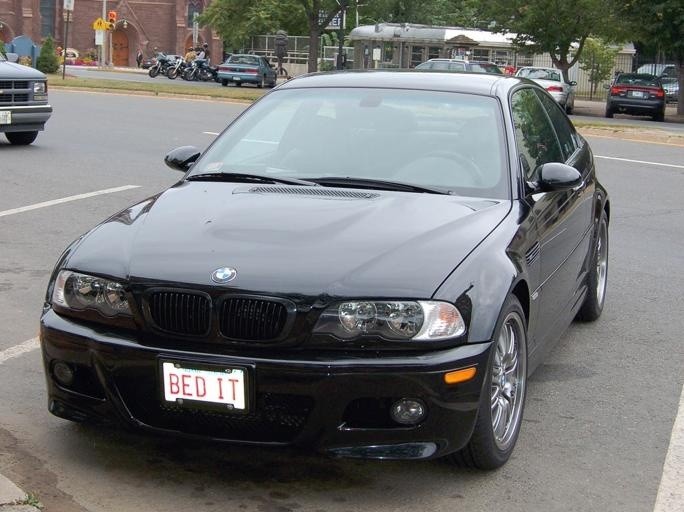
[149,56,222,83]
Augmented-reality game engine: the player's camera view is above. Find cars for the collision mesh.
[61,48,79,60]
[40,68,610,469]
[414,58,679,122]
[0,53,53,144]
[143,55,183,69]
[216,54,276,88]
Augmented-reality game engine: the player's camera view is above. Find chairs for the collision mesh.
[281,108,499,197]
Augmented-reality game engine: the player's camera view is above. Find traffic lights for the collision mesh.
[108,10,117,32]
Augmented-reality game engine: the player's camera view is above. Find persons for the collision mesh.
[184,46,197,63]
[153,47,160,57]
[138,49,143,69]
[56,47,62,56]
[194,42,203,53]
[197,43,210,59]
